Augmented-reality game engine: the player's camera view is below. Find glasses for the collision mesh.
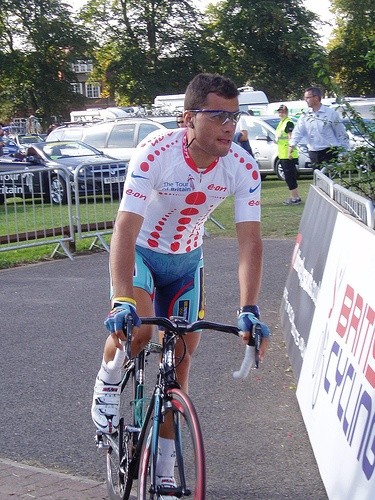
[188,110,242,126]
[304,95,316,99]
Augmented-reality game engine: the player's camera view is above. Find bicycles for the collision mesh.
[93,316,263,500]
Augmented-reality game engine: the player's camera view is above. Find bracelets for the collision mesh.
[111,297,137,307]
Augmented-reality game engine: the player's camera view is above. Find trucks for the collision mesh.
[0,84,375,182]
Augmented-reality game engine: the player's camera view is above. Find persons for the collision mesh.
[274,105,302,206]
[288,86,351,182]
[88,71,276,500]
[176,114,186,129]
[232,129,256,161]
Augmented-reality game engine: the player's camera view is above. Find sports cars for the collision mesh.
[0,141,129,205]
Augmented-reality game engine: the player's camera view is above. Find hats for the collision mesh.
[277,104,288,111]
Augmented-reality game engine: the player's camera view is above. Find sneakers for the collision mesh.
[90,369,122,435]
[153,475,180,500]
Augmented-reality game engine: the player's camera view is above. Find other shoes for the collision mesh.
[282,197,302,206]
